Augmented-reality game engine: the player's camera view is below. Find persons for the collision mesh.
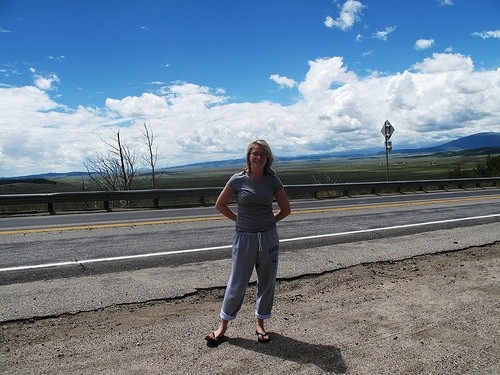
[205,140,291,344]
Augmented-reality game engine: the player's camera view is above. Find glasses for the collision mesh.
[250,152,267,156]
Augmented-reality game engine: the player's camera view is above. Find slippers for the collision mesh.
[205,332,223,343]
[256,330,269,342]
